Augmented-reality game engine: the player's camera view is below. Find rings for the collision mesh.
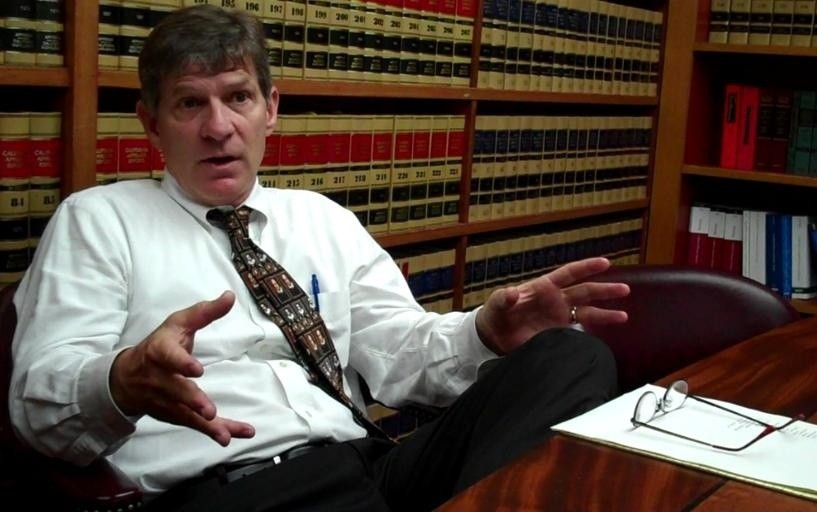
[570,304,578,323]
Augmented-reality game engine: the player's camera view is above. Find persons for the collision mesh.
[7,3,631,512]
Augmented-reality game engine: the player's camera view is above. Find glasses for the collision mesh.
[631,380,805,451]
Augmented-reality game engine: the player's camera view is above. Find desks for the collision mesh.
[412,318,816,509]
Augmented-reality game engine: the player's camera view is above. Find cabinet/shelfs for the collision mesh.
[1,0,667,419]
[648,2,811,309]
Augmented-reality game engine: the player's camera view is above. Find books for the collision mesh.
[356,371,437,441]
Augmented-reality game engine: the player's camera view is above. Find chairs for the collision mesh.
[559,264,793,384]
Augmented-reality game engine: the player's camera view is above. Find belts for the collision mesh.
[226,442,316,487]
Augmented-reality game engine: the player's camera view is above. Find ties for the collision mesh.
[206,205,403,447]
